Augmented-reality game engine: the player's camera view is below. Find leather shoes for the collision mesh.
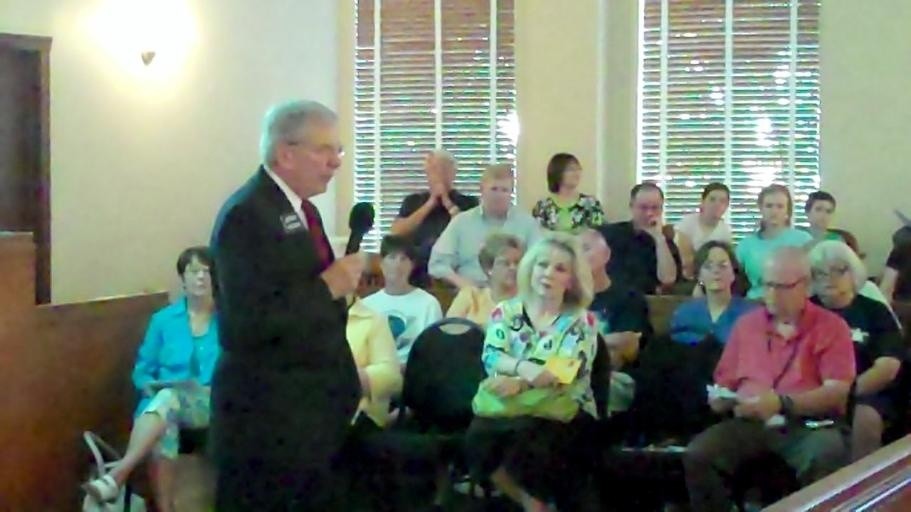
[82,474,118,504]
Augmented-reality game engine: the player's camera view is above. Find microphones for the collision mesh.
[345,202,375,257]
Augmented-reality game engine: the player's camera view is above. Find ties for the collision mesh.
[301,200,333,269]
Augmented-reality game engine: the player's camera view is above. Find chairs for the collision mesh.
[425,289,457,317]
[124,420,210,512]
[590,331,611,421]
[608,323,747,510]
[645,294,690,336]
[385,316,493,510]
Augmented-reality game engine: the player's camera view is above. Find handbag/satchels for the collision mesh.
[81,431,148,512]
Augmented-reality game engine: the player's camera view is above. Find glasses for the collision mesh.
[812,266,850,283]
[760,279,802,291]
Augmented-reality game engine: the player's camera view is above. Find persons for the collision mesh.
[203,97,386,511]
[341,149,910,511]
[81,245,221,509]
